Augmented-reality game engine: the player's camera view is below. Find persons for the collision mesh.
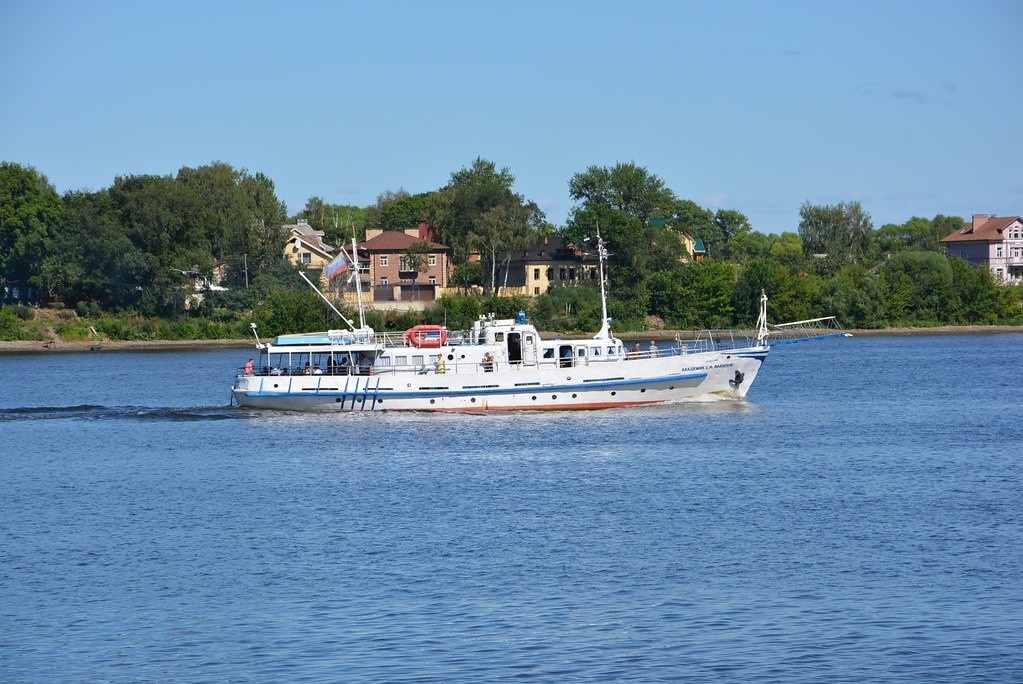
[327,356,350,373]
[245,359,254,374]
[648,340,660,358]
[437,354,445,374]
[263,364,287,374]
[305,362,322,374]
[485,352,494,372]
[295,367,301,373]
[360,354,372,370]
[630,342,640,360]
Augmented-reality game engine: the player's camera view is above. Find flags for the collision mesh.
[324,251,349,279]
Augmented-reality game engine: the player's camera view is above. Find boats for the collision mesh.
[227,214,849,413]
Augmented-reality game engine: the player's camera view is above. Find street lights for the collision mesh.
[244,254,249,289]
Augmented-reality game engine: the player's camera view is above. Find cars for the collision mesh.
[183,285,207,293]
